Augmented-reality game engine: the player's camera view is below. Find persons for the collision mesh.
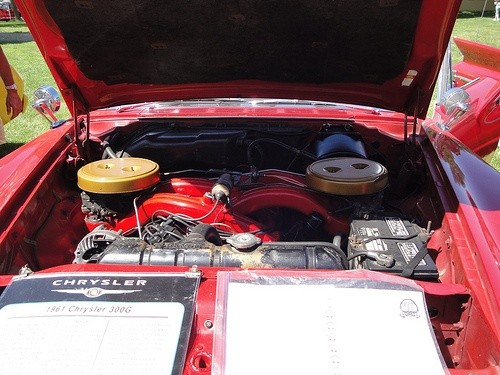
[0,46,23,146]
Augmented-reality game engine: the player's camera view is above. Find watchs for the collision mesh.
[5,82,17,90]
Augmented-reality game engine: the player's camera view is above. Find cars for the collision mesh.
[0,0,500,375]
